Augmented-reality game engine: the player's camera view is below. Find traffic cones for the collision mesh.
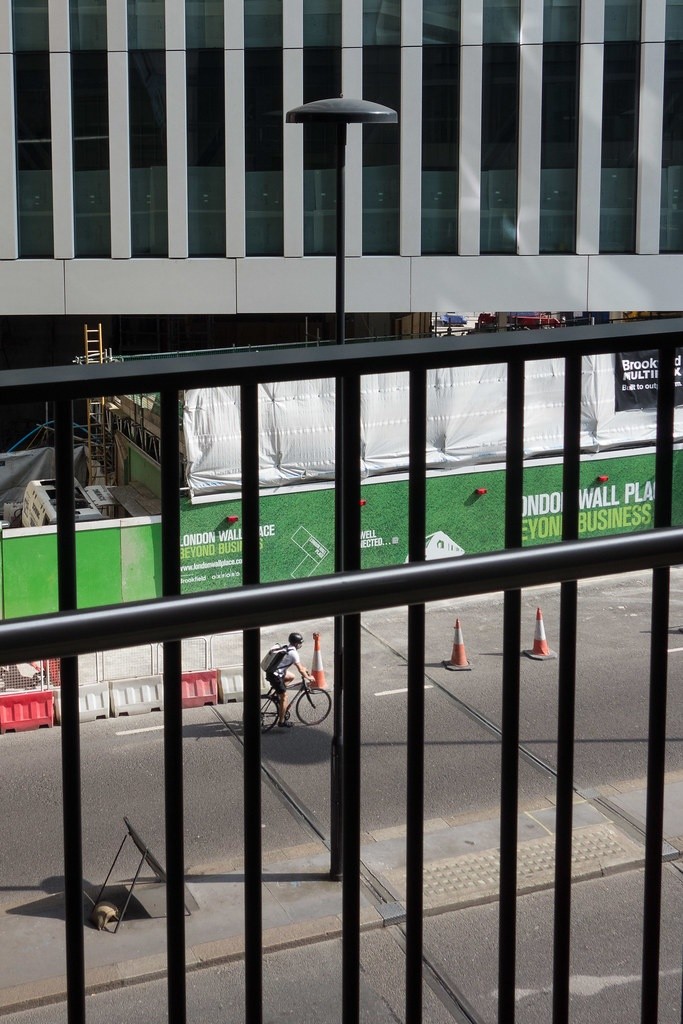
[522,607,559,661]
[304,631,333,694]
[442,617,475,671]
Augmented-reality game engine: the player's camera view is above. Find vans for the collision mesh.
[21,475,103,527]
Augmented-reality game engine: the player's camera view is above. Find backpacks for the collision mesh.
[260,642,296,674]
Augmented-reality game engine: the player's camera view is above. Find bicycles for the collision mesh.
[260,671,334,735]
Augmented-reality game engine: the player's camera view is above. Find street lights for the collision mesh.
[281,97,400,878]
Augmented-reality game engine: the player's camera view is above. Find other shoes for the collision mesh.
[278,720,293,727]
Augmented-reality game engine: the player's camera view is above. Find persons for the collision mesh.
[266,633,316,727]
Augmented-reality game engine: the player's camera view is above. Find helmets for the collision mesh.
[288,632,303,643]
